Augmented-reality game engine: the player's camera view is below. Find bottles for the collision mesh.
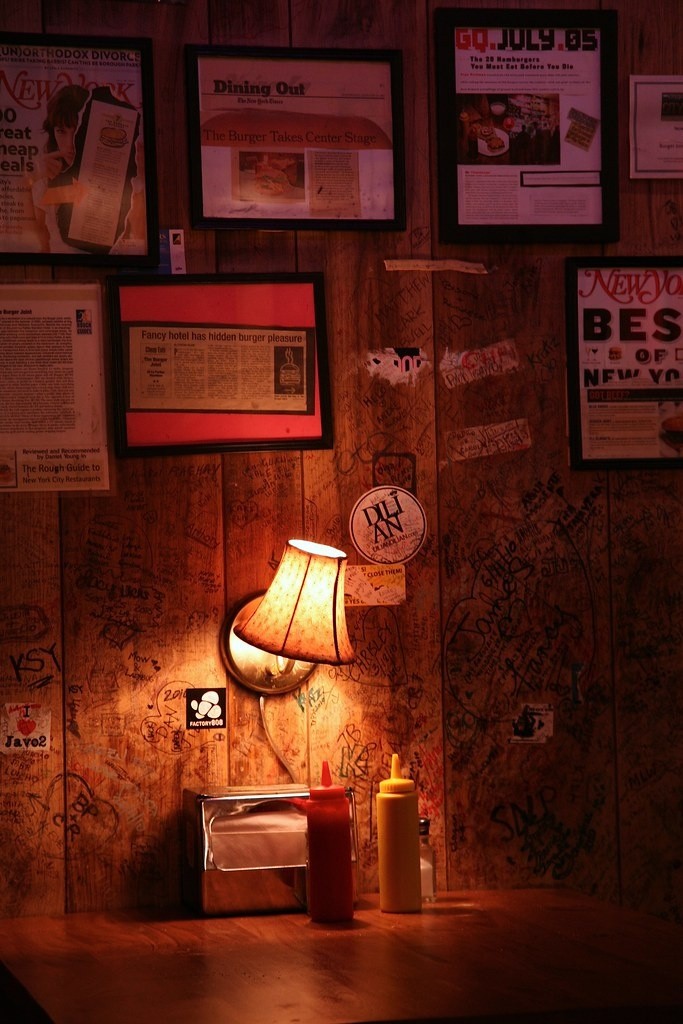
[417,817,436,904]
[375,753,424,913]
[306,761,355,922]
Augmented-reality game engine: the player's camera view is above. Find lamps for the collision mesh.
[218,536,356,694]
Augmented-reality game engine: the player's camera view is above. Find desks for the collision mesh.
[0,889,682,1024]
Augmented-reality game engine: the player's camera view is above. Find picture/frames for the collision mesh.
[562,256,682,470]
[0,31,160,270]
[184,43,407,228]
[105,271,334,453]
[433,8,621,245]
